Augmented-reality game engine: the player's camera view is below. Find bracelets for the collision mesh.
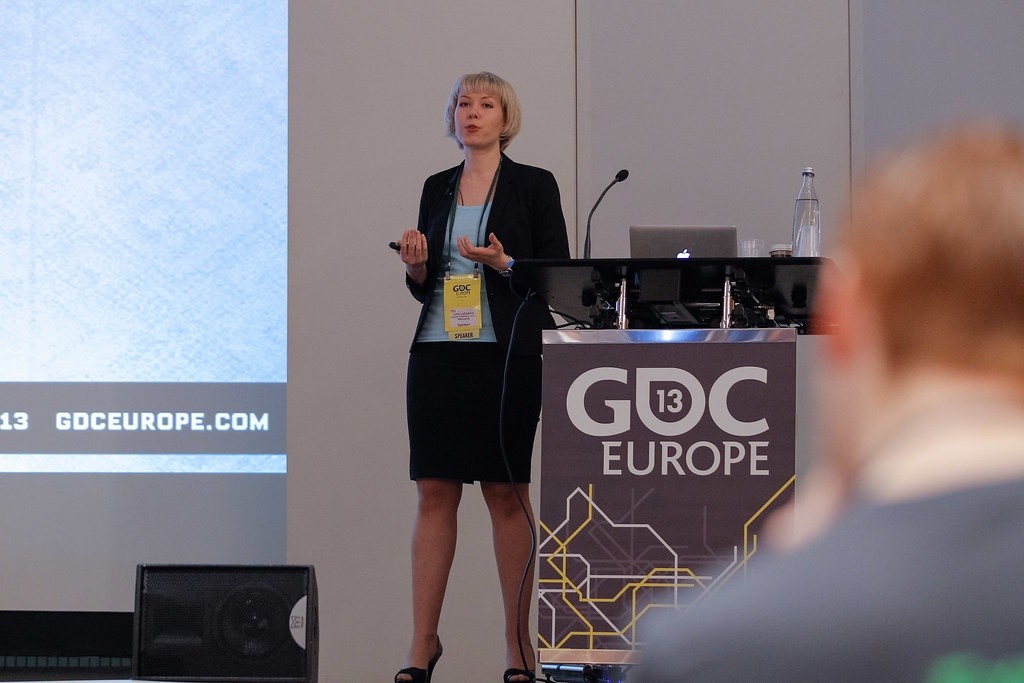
[499,256,513,277]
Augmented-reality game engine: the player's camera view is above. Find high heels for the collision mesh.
[395,634,443,683]
[503,643,535,683]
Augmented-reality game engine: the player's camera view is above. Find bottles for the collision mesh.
[790,167,820,258]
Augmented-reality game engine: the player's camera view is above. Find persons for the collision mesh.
[628,121,1024,683]
[394,71,571,683]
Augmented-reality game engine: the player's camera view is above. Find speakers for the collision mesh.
[134,562,316,683]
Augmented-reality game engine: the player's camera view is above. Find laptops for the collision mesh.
[629,226,738,258]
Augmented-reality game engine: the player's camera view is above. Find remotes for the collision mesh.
[388,242,416,251]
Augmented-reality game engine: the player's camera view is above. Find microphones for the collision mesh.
[582,168,632,262]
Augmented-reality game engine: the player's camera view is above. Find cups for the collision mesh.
[738,238,767,257]
[769,243,792,256]
